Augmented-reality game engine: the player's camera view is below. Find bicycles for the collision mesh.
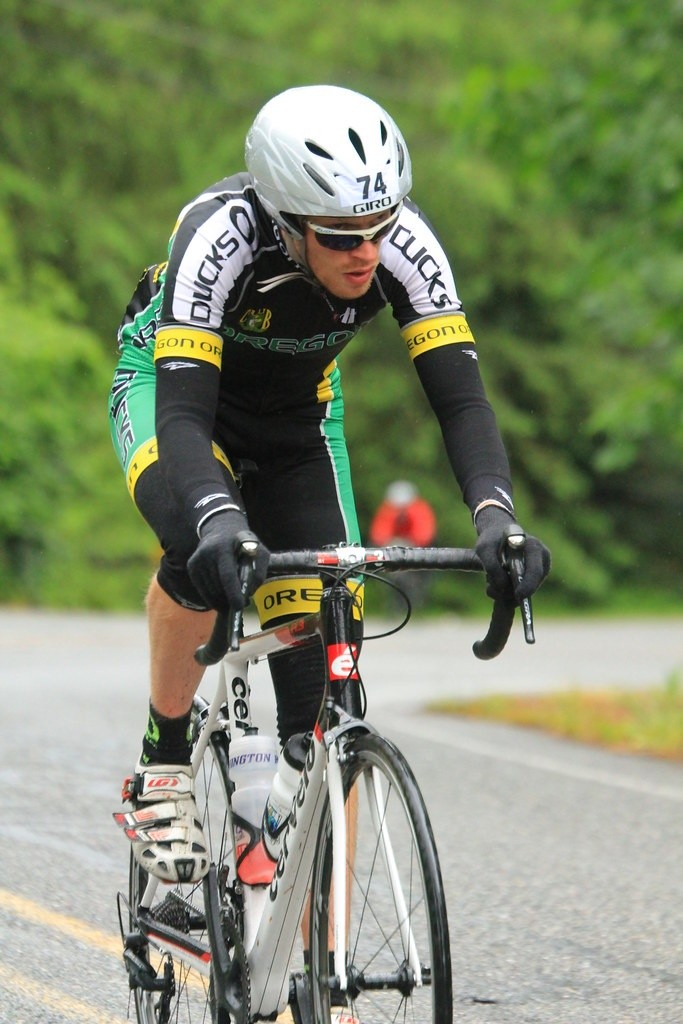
[111,520,536,1024]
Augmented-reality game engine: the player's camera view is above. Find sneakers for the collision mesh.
[113,749,209,881]
[330,1006,359,1024]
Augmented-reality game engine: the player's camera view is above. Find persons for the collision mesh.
[110,83,551,1024]
[369,482,443,600]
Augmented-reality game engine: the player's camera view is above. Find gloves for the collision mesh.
[186,510,270,614]
[474,505,552,603]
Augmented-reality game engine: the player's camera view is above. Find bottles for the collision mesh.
[260,731,315,861]
[227,724,280,886]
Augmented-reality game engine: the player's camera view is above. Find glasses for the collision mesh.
[303,200,404,251]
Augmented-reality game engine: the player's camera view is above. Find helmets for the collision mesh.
[245,84,412,239]
